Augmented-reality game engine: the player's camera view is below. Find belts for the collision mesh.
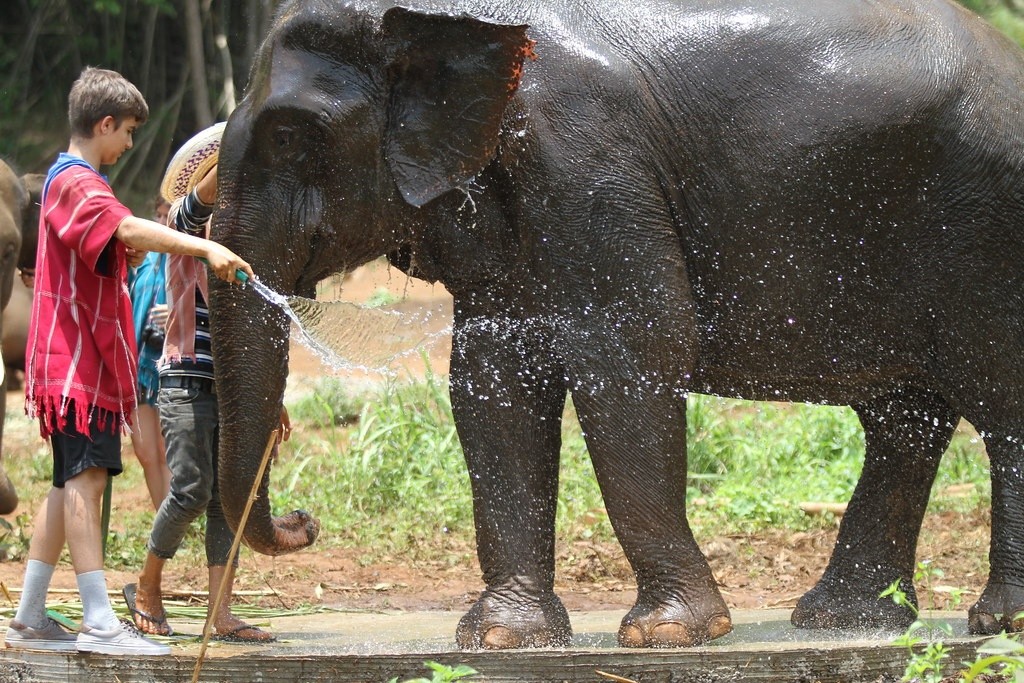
[160,374,216,393]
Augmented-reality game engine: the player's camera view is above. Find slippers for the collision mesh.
[199,626,277,643]
[122,583,173,637]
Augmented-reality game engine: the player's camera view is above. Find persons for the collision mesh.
[126,192,172,513]
[122,120,292,642]
[4,67,256,656]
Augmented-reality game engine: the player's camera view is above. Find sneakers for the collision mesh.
[4,619,77,650]
[76,620,171,655]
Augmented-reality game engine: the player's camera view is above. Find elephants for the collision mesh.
[203,0,1024,655]
[1,156,48,516]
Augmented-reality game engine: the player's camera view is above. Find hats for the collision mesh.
[160,121,227,206]
